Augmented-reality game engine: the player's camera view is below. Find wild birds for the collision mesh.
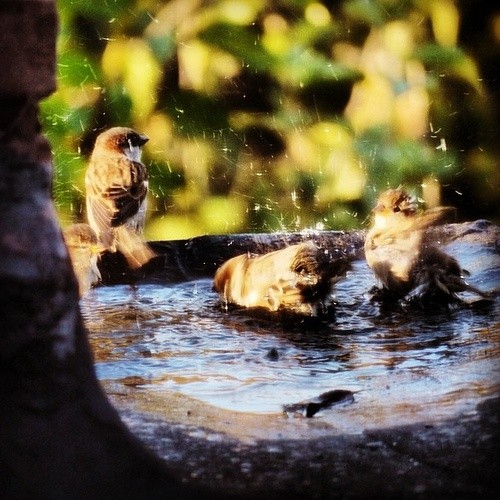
[84,126,156,269]
